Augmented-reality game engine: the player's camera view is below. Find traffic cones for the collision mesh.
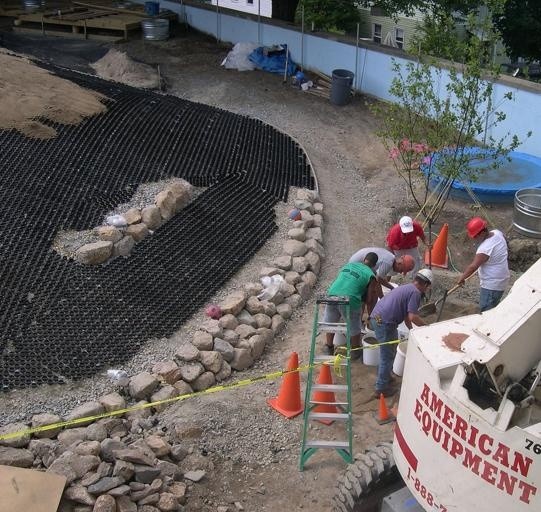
[266,352,304,419]
[373,393,396,426]
[424,223,448,268]
[307,364,341,426]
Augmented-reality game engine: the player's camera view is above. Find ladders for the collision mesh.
[298,299,352,472]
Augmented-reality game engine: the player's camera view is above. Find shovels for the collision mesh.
[418,270,479,315]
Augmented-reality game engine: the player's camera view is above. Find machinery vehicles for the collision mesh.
[333,257,541,512]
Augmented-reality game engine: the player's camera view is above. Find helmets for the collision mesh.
[467,218,486,237]
[418,269,433,284]
[403,255,416,276]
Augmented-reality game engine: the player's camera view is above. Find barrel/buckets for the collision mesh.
[393,341,407,376]
[361,331,379,365]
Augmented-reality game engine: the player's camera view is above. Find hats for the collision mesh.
[399,216,414,233]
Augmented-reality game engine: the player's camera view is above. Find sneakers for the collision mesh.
[350,346,361,360]
[323,344,334,355]
[375,377,396,399]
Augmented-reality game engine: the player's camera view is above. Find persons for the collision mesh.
[323,252,379,361]
[349,246,415,324]
[459,217,512,314]
[371,268,434,400]
[387,215,429,281]
